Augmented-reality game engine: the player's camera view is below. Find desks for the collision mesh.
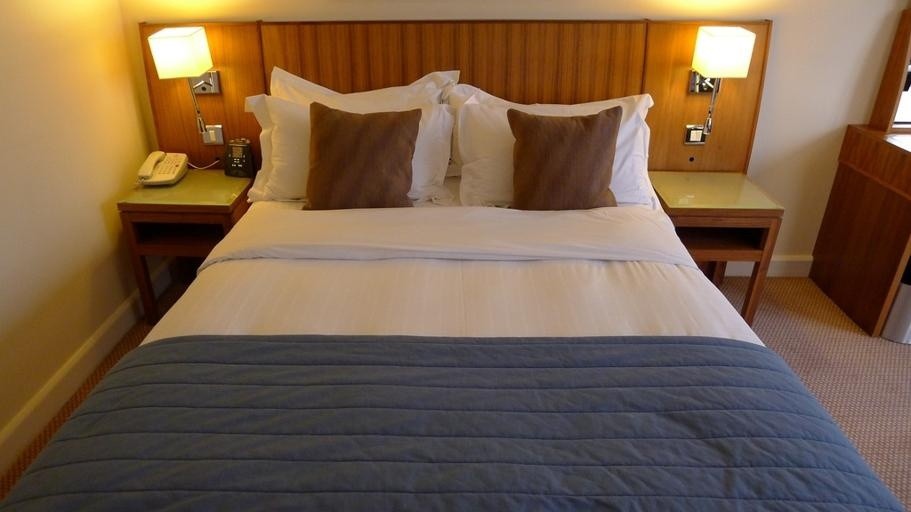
[643,165,786,343]
[118,164,256,320]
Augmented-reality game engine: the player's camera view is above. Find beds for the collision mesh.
[2,17,910,509]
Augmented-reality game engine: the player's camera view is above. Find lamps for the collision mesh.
[145,25,219,136]
[692,25,758,136]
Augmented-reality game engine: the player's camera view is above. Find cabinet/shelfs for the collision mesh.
[809,5,909,341]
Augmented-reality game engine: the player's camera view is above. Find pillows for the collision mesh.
[266,64,462,96]
[243,82,442,207]
[501,105,625,212]
[451,94,656,211]
[304,99,422,210]
[442,83,529,179]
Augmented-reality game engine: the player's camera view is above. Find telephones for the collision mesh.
[137,150,189,186]
